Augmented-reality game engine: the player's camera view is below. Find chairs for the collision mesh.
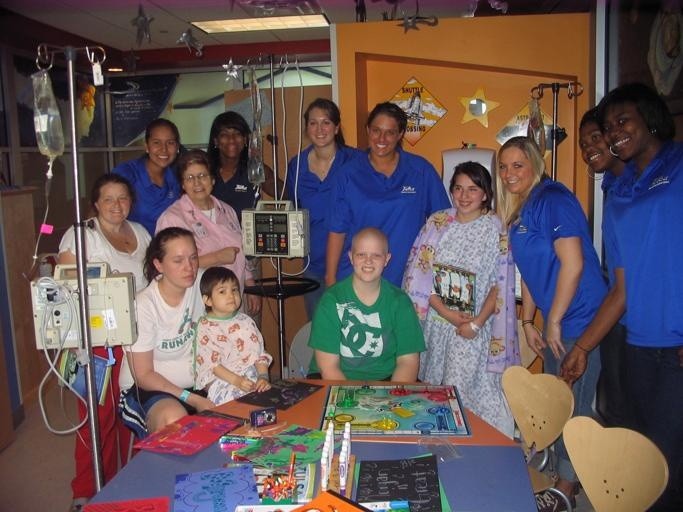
[562,416,670,512]
[501,365,576,512]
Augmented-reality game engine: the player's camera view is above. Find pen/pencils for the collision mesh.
[320,420,350,494]
[220,436,258,449]
[288,453,295,483]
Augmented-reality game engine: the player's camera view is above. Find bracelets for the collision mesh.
[469,321,482,335]
[521,319,534,326]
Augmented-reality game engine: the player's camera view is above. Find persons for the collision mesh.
[195,263,279,409]
[322,100,453,290]
[561,83,681,512]
[117,225,211,446]
[59,170,155,512]
[147,149,267,324]
[400,160,523,441]
[279,97,365,313]
[102,114,193,226]
[204,108,292,225]
[305,227,428,383]
[494,135,609,512]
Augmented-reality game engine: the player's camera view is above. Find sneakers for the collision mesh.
[534,490,576,512]
